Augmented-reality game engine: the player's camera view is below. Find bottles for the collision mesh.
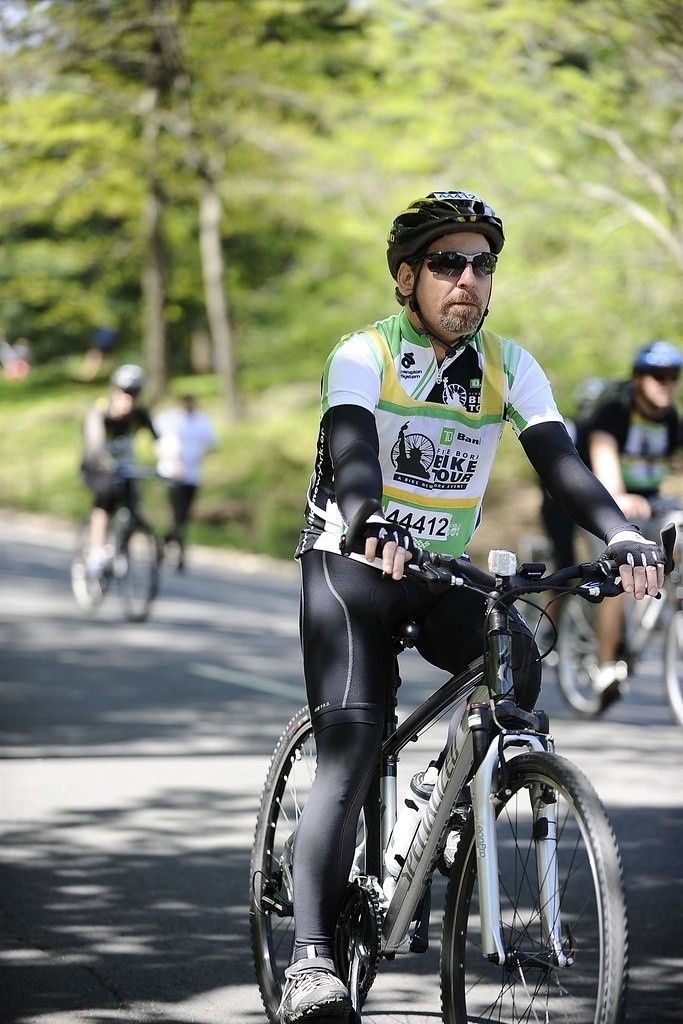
[381,766,442,878]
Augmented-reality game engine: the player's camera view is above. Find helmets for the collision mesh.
[385,190,506,283]
[632,340,683,381]
[110,363,148,390]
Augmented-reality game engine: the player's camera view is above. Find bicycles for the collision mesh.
[553,510,682,730]
[70,459,171,626]
[249,525,664,1023]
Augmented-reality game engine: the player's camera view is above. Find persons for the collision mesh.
[275,191,669,1023]
[152,389,221,576]
[539,341,683,699]
[80,363,162,581]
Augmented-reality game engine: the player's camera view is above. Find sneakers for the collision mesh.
[404,772,472,878]
[275,957,352,1024]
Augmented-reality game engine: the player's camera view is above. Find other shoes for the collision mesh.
[593,662,626,703]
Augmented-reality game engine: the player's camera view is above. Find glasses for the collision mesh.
[407,250,498,278]
